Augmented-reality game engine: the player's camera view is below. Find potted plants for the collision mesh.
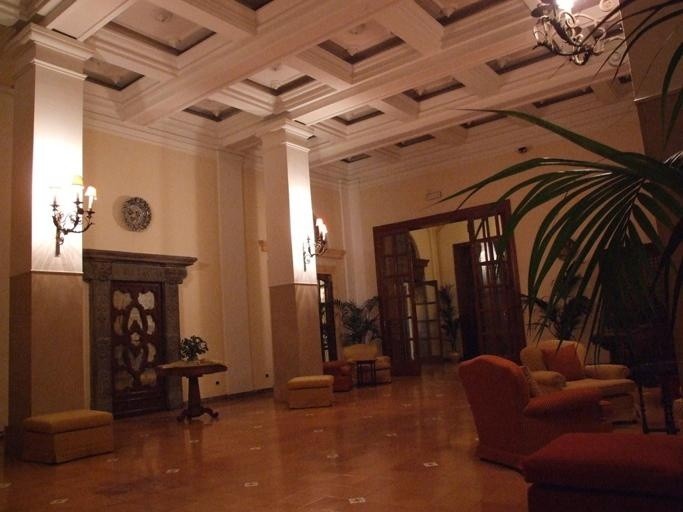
[175,334,208,365]
[437,279,461,364]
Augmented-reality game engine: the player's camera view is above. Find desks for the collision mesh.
[156,360,227,423]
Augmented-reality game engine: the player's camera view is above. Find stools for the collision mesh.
[286,375,334,409]
[21,408,114,465]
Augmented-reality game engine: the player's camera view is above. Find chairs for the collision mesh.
[456,338,683,510]
[322,344,392,392]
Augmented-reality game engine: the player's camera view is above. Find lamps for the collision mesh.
[301,216,329,271]
[53,186,98,257]
